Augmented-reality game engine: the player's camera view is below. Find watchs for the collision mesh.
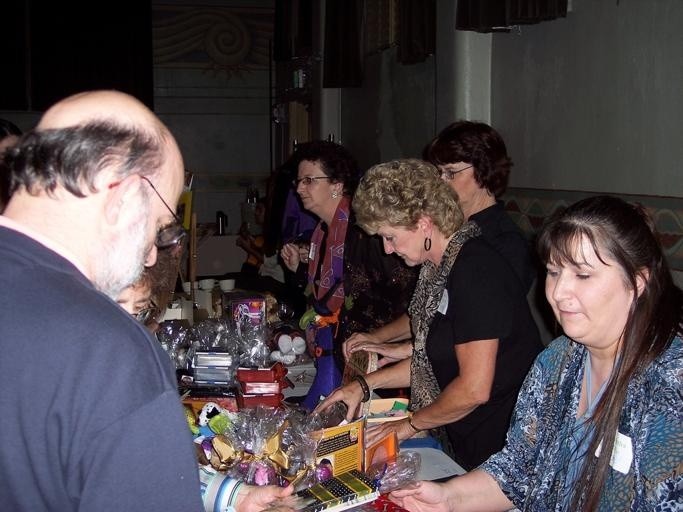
[386,194,682,512]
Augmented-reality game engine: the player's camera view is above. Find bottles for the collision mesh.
[216,211,228,236]
[240,185,259,235]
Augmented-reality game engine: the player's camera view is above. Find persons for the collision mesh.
[309,158,545,473]
[235,182,319,319]
[0,90,206,511]
[113,244,293,511]
[341,119,537,370]
[0,118,23,215]
[279,141,417,375]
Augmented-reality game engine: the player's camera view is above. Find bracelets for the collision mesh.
[405,418,420,434]
[351,375,370,403]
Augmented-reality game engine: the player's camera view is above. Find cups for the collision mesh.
[219,279,235,292]
[182,281,200,295]
[200,279,220,289]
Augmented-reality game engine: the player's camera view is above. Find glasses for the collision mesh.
[139,174,189,249]
[291,176,333,189]
[127,297,161,326]
[436,165,473,181]
